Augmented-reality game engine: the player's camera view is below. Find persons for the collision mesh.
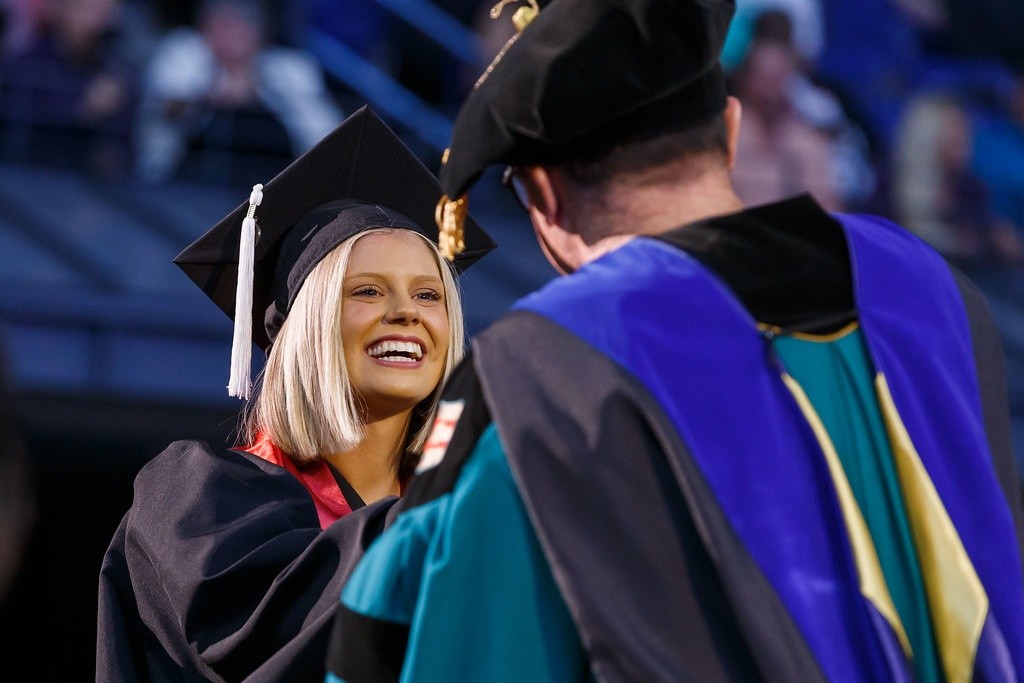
[0,2,1024,273]
[323,2,1023,683]
[91,104,494,683]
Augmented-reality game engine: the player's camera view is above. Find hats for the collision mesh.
[170,104,499,400]
[437,1,738,262]
